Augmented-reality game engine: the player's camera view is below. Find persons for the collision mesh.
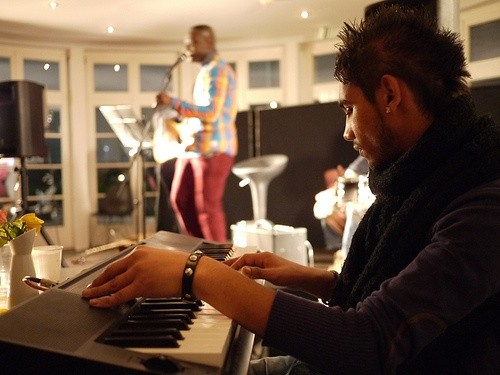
[79,3,500,375]
[151,24,238,245]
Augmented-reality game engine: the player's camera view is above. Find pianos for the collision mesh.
[0,230,265,375]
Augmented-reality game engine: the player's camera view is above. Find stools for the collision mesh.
[231,153,289,219]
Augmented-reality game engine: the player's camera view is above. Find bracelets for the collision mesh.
[180,248,207,301]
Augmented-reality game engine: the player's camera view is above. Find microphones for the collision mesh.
[167,53,188,77]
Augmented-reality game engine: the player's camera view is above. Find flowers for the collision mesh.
[0,210,44,246]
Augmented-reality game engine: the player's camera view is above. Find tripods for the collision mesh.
[10,157,71,269]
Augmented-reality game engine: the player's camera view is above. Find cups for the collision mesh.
[32,245,64,287]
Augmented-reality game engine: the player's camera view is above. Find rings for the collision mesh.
[256,250,261,253]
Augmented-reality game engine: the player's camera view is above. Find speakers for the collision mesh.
[0,79,48,159]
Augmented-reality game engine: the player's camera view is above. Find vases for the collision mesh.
[7,227,40,310]
